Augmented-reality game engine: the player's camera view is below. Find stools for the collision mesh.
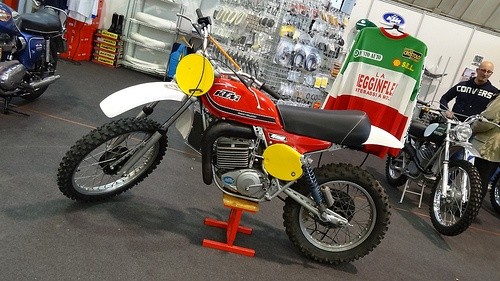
[398,177,426,209]
[202,195,259,256]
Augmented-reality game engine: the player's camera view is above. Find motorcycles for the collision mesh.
[385,99,500,236]
[0,0,71,115]
[489,170,500,215]
[56,7,392,264]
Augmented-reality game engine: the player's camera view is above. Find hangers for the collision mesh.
[384,24,404,35]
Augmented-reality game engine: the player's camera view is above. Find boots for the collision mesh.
[114,15,124,34]
[108,14,118,32]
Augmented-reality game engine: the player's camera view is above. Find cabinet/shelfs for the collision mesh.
[116,0,185,82]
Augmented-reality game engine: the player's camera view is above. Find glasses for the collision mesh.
[479,69,493,74]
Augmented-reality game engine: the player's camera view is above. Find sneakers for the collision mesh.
[455,211,481,223]
[418,177,427,187]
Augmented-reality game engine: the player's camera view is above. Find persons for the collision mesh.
[462,94,497,228]
[441,62,497,188]
